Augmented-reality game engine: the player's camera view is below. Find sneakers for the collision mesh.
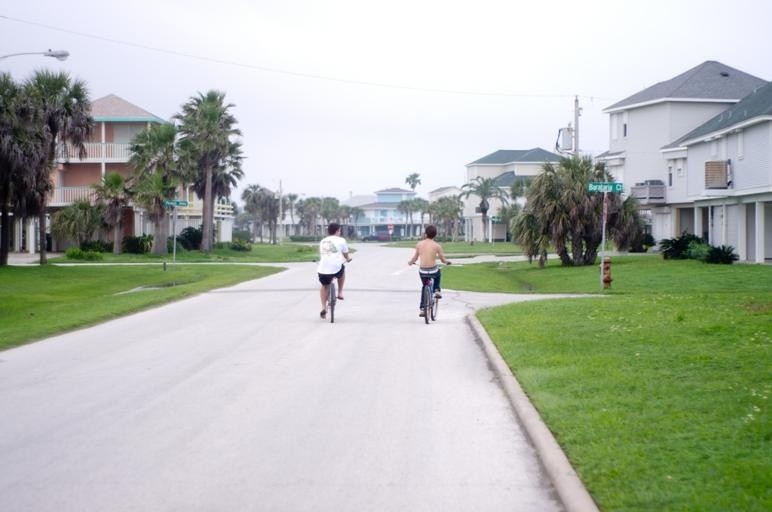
[435,291,442,299]
[418,311,425,317]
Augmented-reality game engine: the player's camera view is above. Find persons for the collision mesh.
[406,224,452,318]
[316,221,353,319]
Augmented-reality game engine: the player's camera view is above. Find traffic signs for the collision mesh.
[163,199,190,208]
[588,181,624,195]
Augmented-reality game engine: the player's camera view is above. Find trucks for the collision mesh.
[361,231,391,242]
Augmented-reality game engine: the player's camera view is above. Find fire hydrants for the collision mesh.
[598,254,615,291]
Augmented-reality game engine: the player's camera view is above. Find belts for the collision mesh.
[420,269,438,274]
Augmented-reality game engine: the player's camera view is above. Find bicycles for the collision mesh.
[412,260,452,324]
[311,258,350,324]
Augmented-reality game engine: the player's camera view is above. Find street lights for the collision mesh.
[1,48,71,62]
